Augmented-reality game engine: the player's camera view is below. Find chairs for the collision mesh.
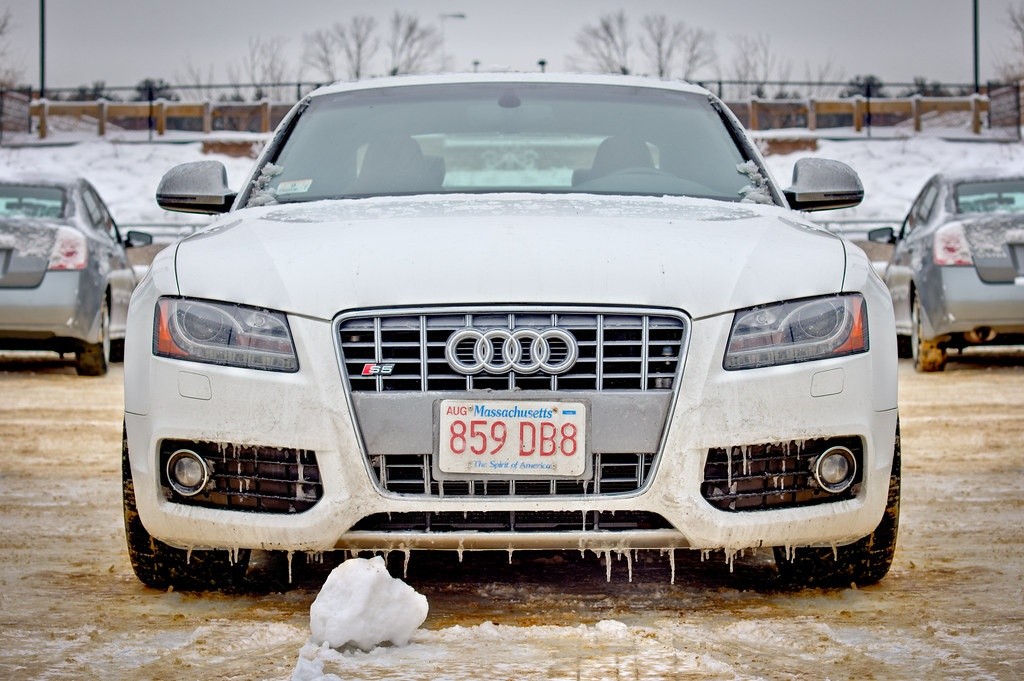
[350,133,663,194]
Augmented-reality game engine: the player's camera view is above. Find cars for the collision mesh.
[868,166,1024,371]
[0,168,152,375]
[119,71,902,594]
